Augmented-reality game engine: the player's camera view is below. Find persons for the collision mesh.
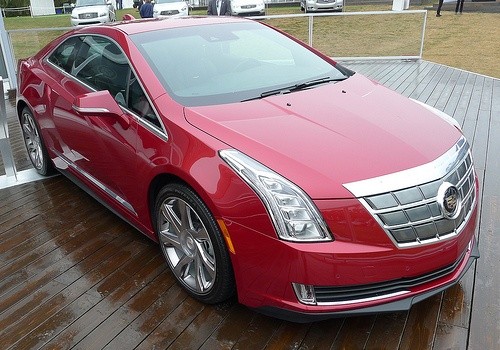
[454,0,464,15]
[207,0,232,16]
[140,0,154,18]
[436,0,444,17]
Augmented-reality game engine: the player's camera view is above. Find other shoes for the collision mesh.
[455,11,464,15]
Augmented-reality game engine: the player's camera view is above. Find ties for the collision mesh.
[217,0,219,7]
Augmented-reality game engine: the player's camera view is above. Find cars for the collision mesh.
[70,0,116,28]
[230,0,266,16]
[152,0,189,18]
[301,0,343,12]
[15,13,481,325]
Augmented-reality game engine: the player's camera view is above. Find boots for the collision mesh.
[436,10,442,17]
